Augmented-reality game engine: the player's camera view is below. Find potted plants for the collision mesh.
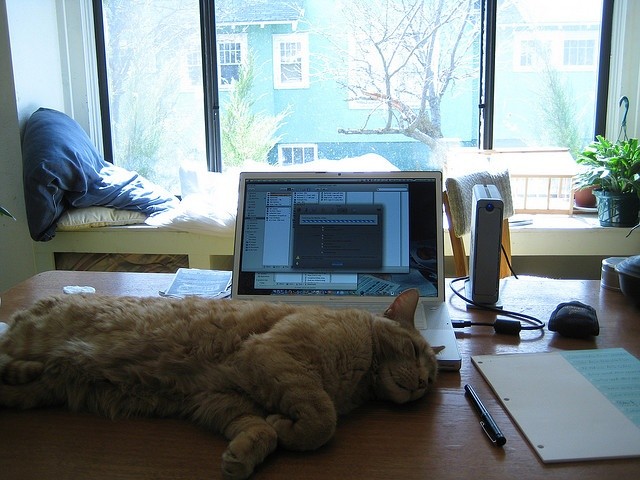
[577,135,640,227]
[570,172,600,208]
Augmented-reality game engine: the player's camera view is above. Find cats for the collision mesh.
[0,283,446,479]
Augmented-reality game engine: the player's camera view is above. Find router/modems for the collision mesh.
[470,184,504,305]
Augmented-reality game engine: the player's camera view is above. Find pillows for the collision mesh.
[57,207,145,231]
[145,155,277,239]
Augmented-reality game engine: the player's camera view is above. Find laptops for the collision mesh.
[230,171,462,371]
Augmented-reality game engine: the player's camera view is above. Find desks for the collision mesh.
[0,270,640,479]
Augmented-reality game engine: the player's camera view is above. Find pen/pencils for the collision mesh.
[464,383,506,447]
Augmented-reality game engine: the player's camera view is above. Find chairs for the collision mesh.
[442,170,512,279]
